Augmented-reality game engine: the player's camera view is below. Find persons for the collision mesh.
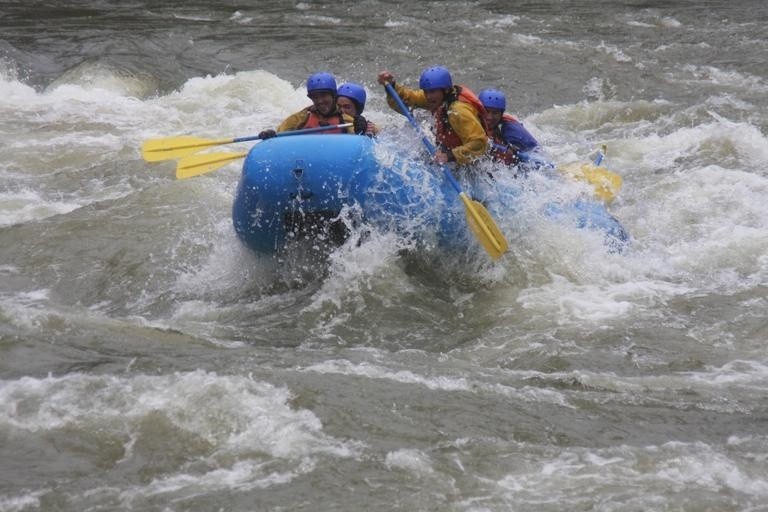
[258,71,367,140]
[336,85,379,138]
[378,67,488,167]
[478,88,542,173]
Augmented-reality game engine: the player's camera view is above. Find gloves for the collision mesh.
[352,116,368,135]
[259,130,276,140]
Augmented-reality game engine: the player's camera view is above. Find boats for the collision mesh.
[233,132,627,275]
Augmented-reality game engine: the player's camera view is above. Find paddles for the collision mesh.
[141,120,353,163]
[490,142,623,203]
[381,76,508,262]
[176,150,251,180]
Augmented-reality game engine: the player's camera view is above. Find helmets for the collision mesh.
[337,83,366,115]
[478,89,506,112]
[307,73,337,97]
[419,67,452,91]
